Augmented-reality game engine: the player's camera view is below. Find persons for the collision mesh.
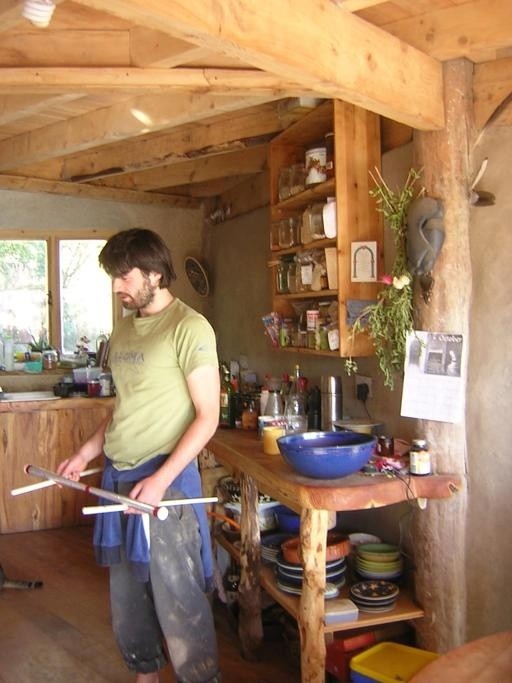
[54,226,221,683]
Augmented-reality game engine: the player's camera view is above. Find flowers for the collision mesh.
[344,161,429,393]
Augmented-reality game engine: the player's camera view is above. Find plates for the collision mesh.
[351,542,405,581]
[333,417,384,428]
[350,579,400,614]
[257,533,297,562]
[271,550,349,596]
[185,255,211,297]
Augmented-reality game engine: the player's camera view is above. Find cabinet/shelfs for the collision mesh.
[270,97,384,360]
[195,414,462,682]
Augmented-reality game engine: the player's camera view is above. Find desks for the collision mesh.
[402,629,512,682]
[1,391,117,532]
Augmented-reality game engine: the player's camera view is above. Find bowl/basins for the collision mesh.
[348,532,382,547]
[274,426,378,478]
[71,366,103,383]
[218,476,277,504]
[271,504,340,533]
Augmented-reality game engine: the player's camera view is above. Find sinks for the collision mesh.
[0,391,61,401]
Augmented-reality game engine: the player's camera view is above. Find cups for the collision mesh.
[87,380,102,397]
[261,425,287,454]
[98,378,111,396]
[257,416,273,437]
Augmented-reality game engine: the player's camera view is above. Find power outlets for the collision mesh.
[355,373,373,399]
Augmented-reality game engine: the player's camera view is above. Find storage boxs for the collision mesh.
[349,639,438,682]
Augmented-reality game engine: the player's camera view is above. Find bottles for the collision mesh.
[218,364,236,426]
[42,349,57,369]
[283,363,307,435]
[270,220,283,249]
[278,212,297,248]
[272,252,296,293]
[241,398,259,430]
[308,197,327,238]
[275,165,291,200]
[320,373,343,432]
[407,439,432,476]
[264,380,285,416]
[289,163,306,194]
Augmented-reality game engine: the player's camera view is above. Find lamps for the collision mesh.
[21,0,59,30]
